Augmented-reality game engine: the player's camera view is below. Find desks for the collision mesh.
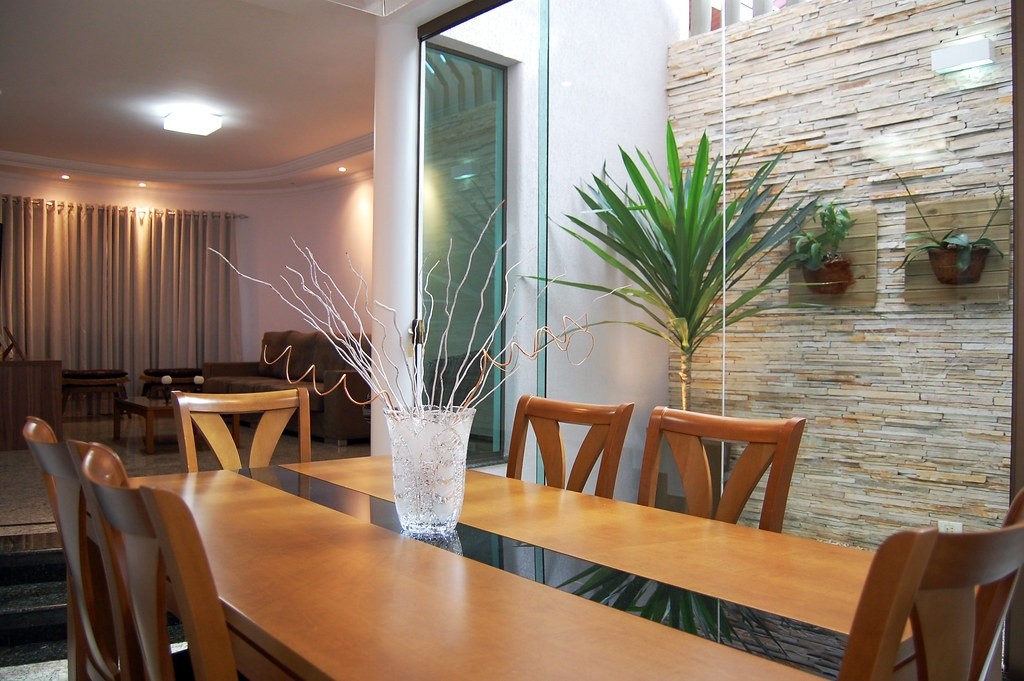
[119,452,980,681]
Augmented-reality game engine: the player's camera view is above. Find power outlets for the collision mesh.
[936,520,963,534]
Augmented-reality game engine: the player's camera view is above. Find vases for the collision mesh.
[383,403,477,535]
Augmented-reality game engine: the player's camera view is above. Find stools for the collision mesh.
[61,367,203,421]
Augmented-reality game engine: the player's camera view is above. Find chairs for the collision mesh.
[504,394,636,499]
[638,403,806,535]
[0,382,313,681]
[835,488,1024,681]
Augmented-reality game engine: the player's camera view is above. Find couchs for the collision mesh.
[202,330,372,447]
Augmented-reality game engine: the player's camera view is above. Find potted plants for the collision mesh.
[781,197,860,297]
[888,166,1007,286]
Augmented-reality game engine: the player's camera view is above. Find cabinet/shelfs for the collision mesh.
[0,360,62,452]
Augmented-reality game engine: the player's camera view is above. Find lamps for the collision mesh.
[160,99,222,138]
[930,38,996,75]
[450,158,482,181]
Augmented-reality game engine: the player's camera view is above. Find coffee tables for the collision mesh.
[111,396,241,455]
